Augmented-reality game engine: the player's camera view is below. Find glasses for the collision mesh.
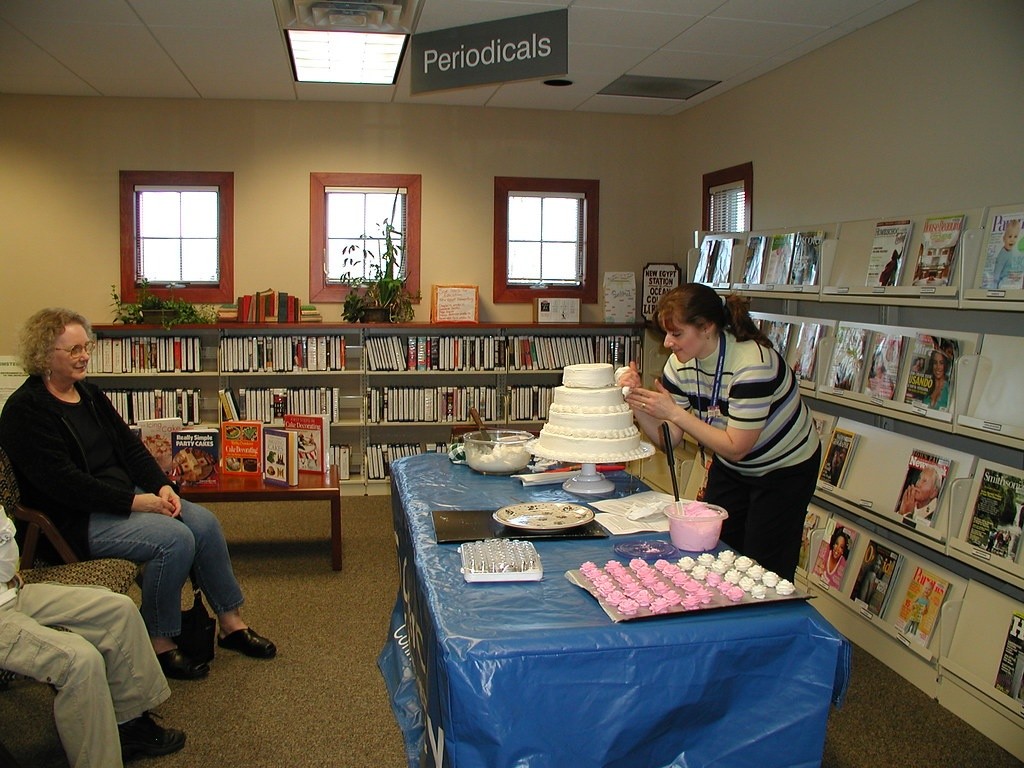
[55,340,95,358]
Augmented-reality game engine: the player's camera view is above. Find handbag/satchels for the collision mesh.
[139,596,215,658]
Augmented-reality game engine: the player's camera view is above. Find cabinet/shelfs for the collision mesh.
[672,288,1024,763]
[78,323,671,498]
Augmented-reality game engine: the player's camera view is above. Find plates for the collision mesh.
[492,501,596,534]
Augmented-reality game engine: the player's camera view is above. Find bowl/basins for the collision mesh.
[463,429,535,475]
[662,502,729,552]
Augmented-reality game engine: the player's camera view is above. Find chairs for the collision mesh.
[0,446,141,679]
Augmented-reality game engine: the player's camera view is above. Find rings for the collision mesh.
[640,403,646,409]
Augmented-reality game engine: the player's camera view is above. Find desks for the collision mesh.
[372,452,853,768]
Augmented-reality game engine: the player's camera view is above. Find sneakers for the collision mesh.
[119,711,186,758]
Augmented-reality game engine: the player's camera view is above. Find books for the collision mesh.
[904,334,960,412]
[692,212,1024,392]
[965,467,1024,563]
[100,387,350,487]
[864,332,911,401]
[87,287,346,373]
[818,425,861,488]
[814,419,826,434]
[365,334,643,479]
[694,459,954,650]
[993,611,1024,705]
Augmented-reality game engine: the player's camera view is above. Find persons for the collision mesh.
[911,332,953,411]
[800,336,816,380]
[859,554,883,602]
[896,465,943,522]
[617,282,822,587]
[986,528,1011,558]
[822,526,850,589]
[0,502,187,768]
[904,581,934,635]
[832,450,845,476]
[0,307,276,679]
[868,340,900,401]
[991,220,1024,289]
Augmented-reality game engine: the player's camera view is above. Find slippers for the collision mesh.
[217,626,277,658]
[158,648,211,680]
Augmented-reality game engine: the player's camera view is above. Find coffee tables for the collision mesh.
[176,464,344,570]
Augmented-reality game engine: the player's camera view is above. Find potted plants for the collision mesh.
[109,277,219,330]
[338,185,424,324]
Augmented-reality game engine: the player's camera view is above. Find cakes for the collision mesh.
[533,363,644,460]
